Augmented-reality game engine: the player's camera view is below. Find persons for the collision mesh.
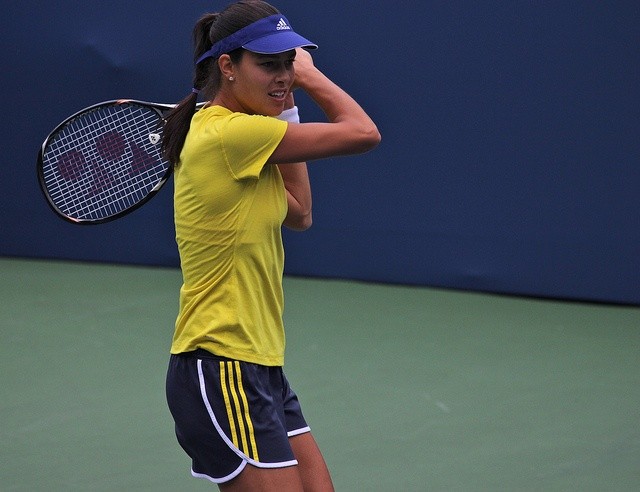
[160,1,385,492]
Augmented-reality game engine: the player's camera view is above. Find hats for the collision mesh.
[196,13,320,65]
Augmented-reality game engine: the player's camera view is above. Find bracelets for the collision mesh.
[270,105,300,124]
